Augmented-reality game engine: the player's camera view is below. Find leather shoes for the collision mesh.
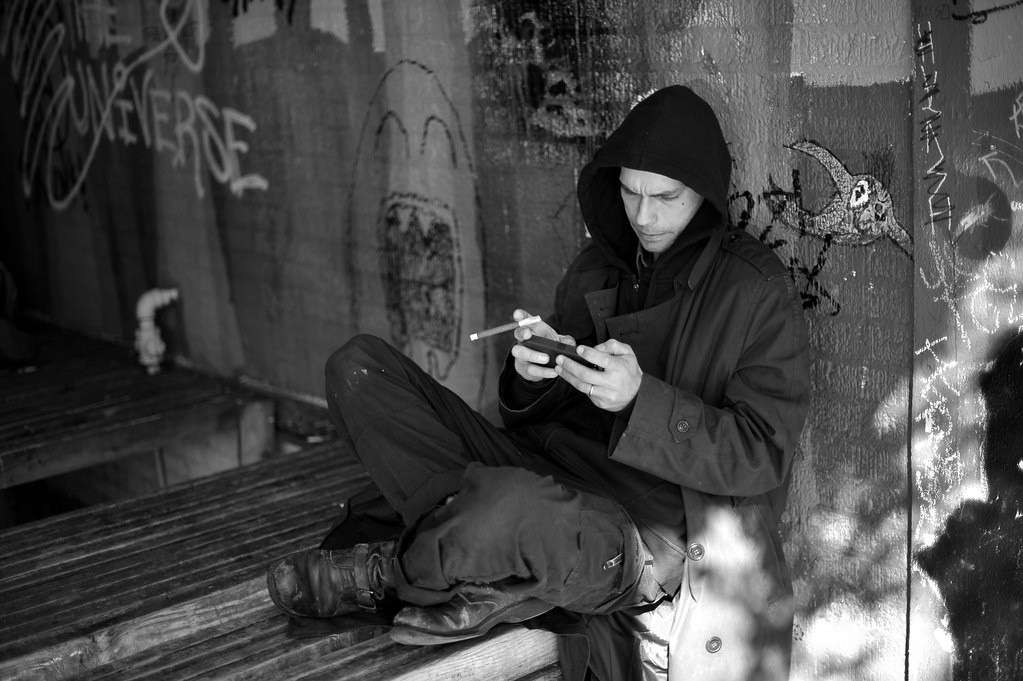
[388,585,557,646]
[266,539,397,618]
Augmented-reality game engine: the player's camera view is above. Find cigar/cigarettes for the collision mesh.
[469,315,541,341]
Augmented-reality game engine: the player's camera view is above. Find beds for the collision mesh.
[0,348,559,681]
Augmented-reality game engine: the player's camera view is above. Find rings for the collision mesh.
[589,385,594,396]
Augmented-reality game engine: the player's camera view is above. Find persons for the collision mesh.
[265,86,811,681]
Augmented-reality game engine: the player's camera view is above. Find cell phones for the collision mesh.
[522,335,604,371]
